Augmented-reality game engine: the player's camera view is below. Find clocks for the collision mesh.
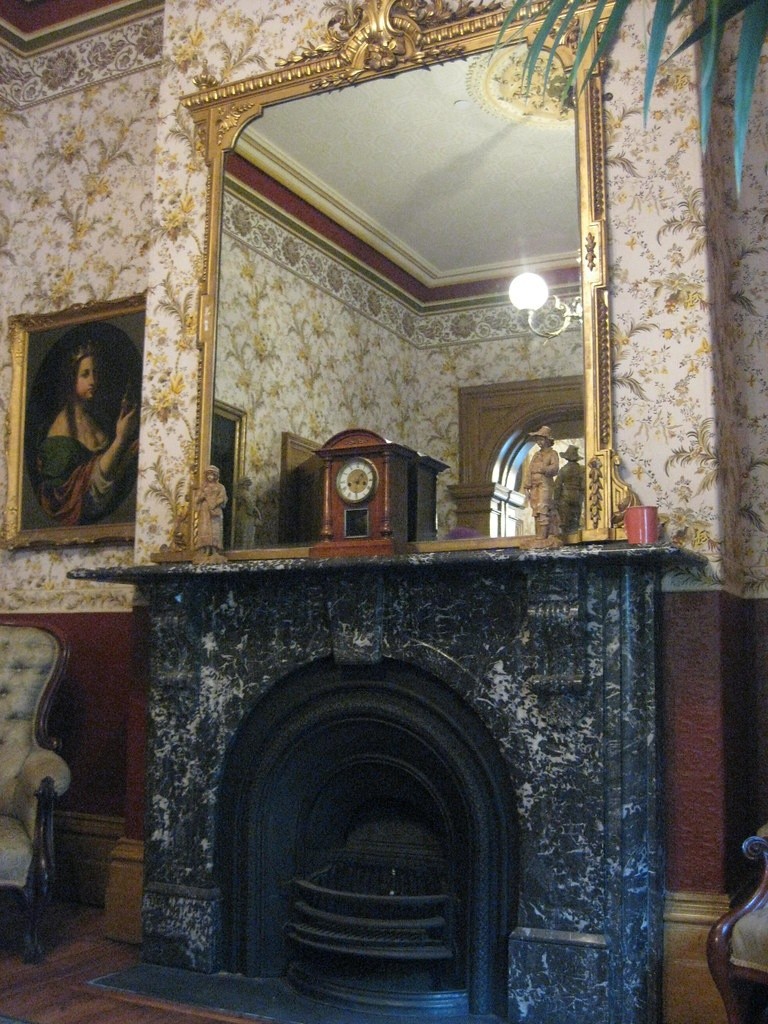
[411,452,451,542]
[309,429,410,557]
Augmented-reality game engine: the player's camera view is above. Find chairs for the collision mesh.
[0,618,71,965]
[706,822,768,1024]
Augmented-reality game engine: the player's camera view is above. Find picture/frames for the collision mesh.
[279,432,325,544]
[212,398,247,550]
[0,286,149,552]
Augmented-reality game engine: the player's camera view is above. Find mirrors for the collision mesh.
[150,0,641,567]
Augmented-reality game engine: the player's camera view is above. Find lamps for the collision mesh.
[509,273,583,340]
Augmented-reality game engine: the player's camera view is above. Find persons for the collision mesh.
[555,445,584,533]
[234,476,263,551]
[523,426,559,550]
[192,465,227,566]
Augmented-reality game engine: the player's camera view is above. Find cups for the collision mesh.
[625,506,658,545]
